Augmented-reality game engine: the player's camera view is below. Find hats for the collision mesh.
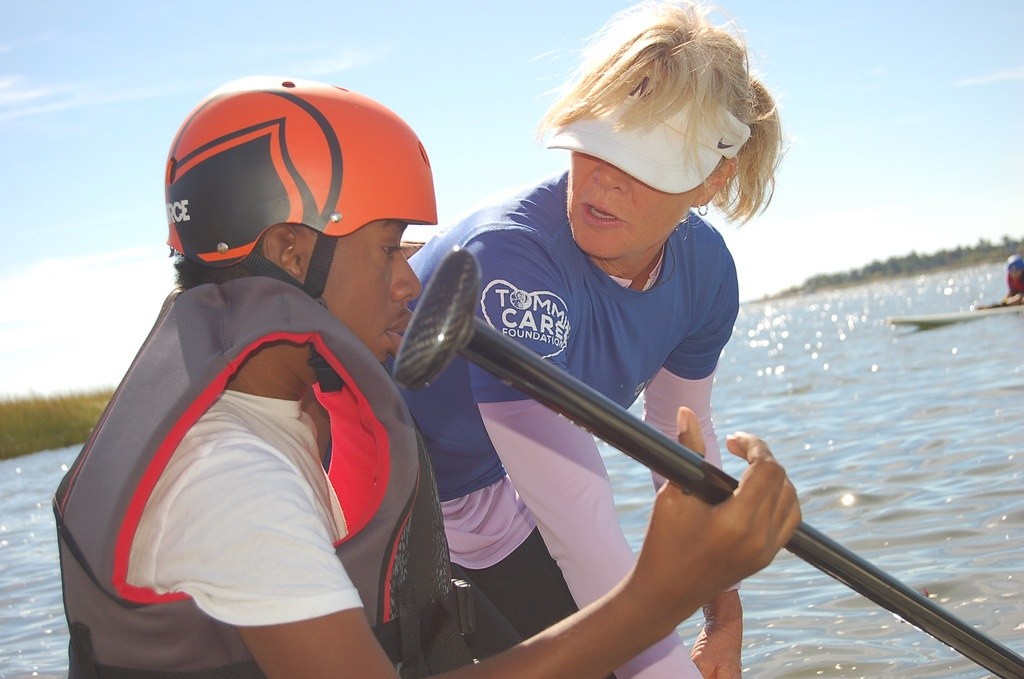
[546,73,751,194]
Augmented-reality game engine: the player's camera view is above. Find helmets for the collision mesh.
[1007,255,1024,271]
[164,76,437,268]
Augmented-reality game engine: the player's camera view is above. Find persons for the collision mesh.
[378,0,783,679]
[49,77,800,679]
[1006,257,1024,302]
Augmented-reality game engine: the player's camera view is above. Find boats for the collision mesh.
[885,285,1023,327]
